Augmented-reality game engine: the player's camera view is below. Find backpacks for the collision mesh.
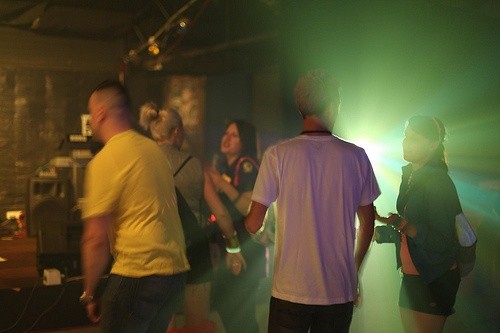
[173,156,213,284]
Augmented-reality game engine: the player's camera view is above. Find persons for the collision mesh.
[80,79,191,333]
[138,99,246,333]
[372,112,477,333]
[206,120,266,333]
[245,69,381,333]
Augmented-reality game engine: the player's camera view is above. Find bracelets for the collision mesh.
[80,291,94,304]
[222,231,241,253]
[392,217,412,234]
[232,192,241,204]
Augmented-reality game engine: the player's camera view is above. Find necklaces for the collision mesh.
[300,130,332,135]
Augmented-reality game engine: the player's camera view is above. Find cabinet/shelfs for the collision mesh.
[28,177,67,237]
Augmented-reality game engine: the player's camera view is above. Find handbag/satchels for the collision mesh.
[233,157,275,245]
[454,206,477,278]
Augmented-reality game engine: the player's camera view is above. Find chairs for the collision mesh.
[33,198,68,276]
